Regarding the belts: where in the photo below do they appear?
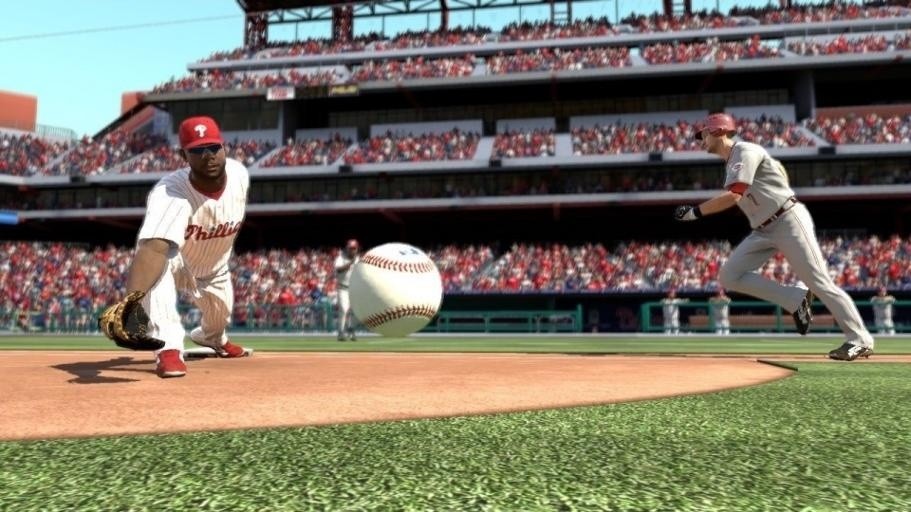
[759,197,797,230]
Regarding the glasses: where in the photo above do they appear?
[189,144,221,154]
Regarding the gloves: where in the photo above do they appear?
[675,206,704,220]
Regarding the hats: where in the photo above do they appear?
[346,240,359,248]
[179,117,224,149]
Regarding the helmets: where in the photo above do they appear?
[694,114,737,138]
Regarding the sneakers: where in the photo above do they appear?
[830,344,872,361]
[157,349,187,376]
[191,335,245,358]
[793,290,813,335]
